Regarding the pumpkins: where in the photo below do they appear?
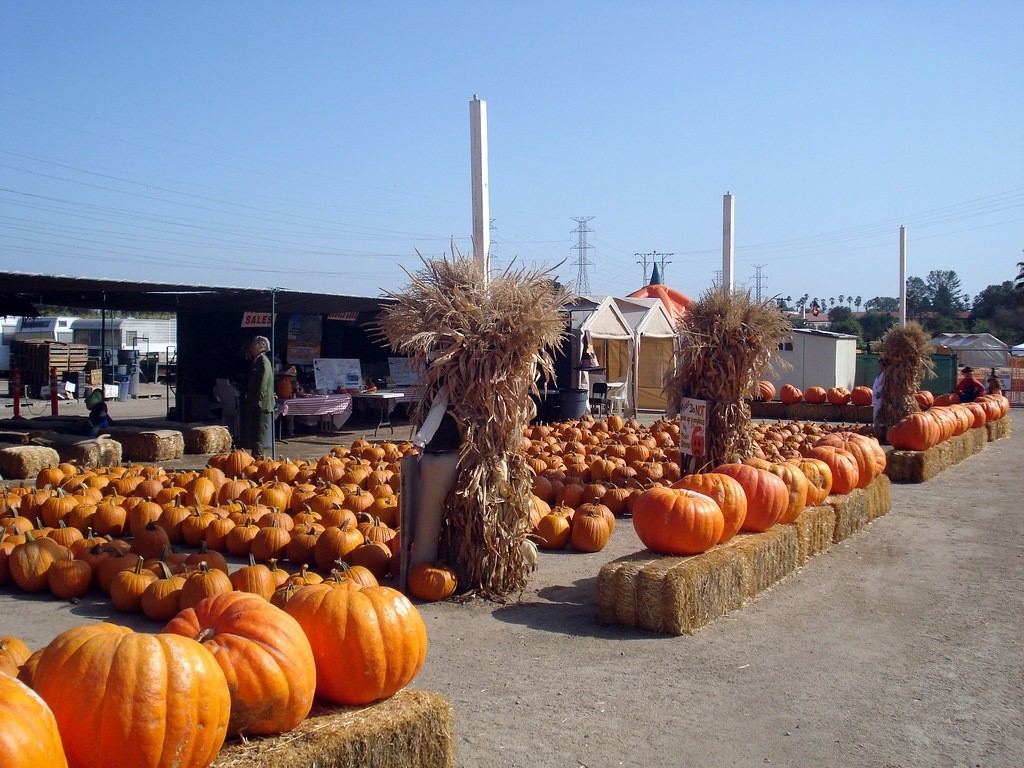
[0,431,459,768]
[522,410,888,554]
[750,379,875,406]
[885,390,1009,450]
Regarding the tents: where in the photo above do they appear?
[928,332,1008,367]
[571,294,679,421]
[1012,343,1024,356]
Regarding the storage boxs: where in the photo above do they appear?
[40,369,120,400]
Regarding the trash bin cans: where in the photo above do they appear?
[108,374,130,402]
[558,388,587,421]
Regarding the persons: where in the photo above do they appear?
[281,365,305,438]
[406,351,460,572]
[245,334,276,460]
[871,353,892,423]
[958,366,984,403]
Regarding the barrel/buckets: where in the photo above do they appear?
[558,388,588,422]
[98,349,139,401]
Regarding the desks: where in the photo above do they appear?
[352,392,404,437]
[275,394,353,444]
[357,388,425,424]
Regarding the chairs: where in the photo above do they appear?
[589,383,609,418]
[214,384,242,440]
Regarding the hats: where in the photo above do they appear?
[256,336,271,352]
[962,367,973,373]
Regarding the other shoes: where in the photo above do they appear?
[288,433,295,438]
